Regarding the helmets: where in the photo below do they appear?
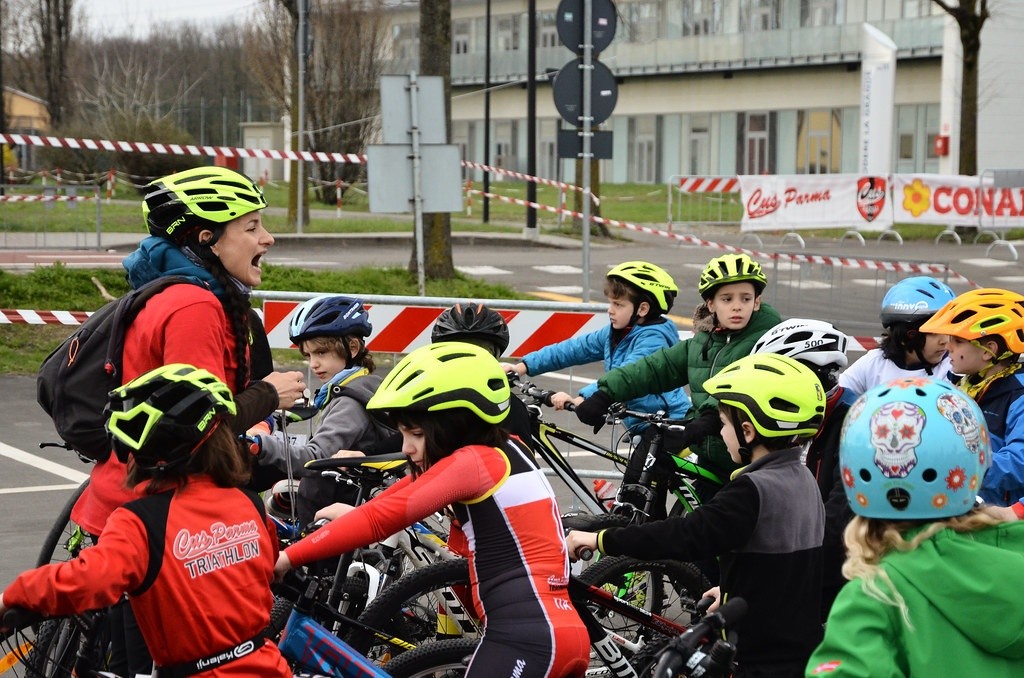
[837,378,994,519]
[287,294,372,344]
[365,342,511,424]
[880,278,956,327]
[917,287,1024,355]
[608,258,678,316]
[703,355,827,440]
[696,255,767,300]
[749,317,847,373]
[431,302,509,353]
[141,167,267,242]
[102,362,240,460]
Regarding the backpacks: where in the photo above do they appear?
[36,273,208,461]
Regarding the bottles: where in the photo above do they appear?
[593,478,618,509]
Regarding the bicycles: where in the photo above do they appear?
[0,370,749,678]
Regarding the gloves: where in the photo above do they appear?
[577,390,613,433]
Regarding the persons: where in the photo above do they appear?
[503,261,692,431]
[575,254,783,502]
[566,317,860,678]
[804,377,1024,678]
[241,297,401,477]
[297,303,533,576]
[69,166,305,678]
[272,342,590,678]
[837,276,1024,520]
[0,363,293,678]
